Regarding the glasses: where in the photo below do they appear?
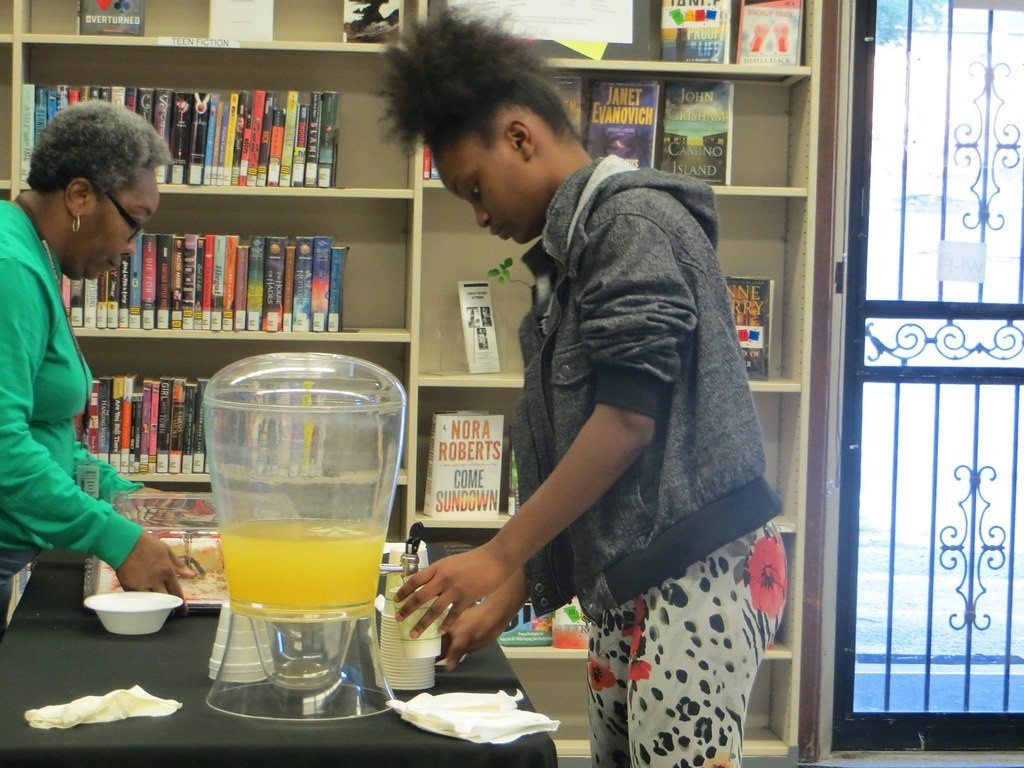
[95,182,146,245]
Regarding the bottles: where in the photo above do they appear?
[552,595,592,649]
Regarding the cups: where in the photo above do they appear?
[377,540,441,690]
[206,602,276,683]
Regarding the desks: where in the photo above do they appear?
[4,534,559,768]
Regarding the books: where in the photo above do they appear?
[1,0,799,664]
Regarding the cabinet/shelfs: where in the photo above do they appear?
[0,1,826,768]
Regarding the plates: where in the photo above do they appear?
[83,535,231,611]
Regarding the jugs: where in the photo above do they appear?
[202,352,405,719]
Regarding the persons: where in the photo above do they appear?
[373,0,788,767]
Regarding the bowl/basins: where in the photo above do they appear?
[83,592,183,635]
